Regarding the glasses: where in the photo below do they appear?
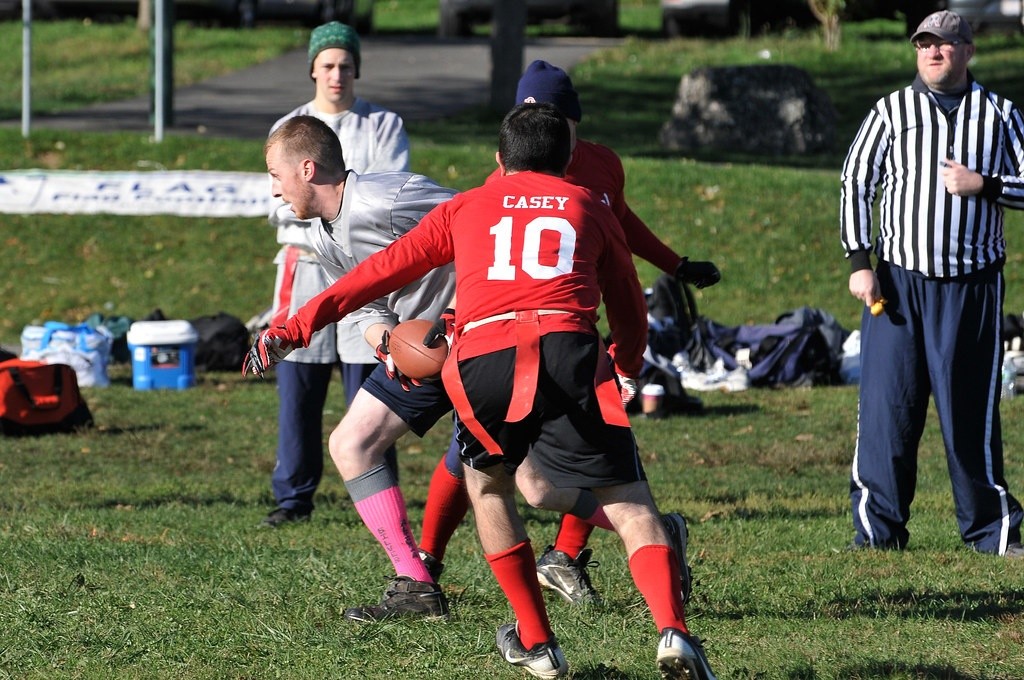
[915,38,968,51]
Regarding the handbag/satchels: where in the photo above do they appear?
[0,309,251,436]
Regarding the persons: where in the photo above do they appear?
[263,115,694,621]
[242,102,717,680]
[839,11,1024,556]
[264,20,410,529]
[413,59,721,605]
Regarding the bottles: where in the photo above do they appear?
[1000,356,1016,399]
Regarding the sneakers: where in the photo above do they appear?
[382,548,444,601]
[536,545,601,603]
[495,619,568,679]
[348,576,450,623]
[655,627,716,680]
[659,512,693,606]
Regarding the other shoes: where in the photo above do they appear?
[261,509,310,530]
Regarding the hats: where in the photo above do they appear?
[308,21,360,84]
[516,60,582,123]
[910,10,972,43]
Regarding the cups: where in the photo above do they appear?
[641,385,664,413]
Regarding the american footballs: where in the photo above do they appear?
[388,318,448,381]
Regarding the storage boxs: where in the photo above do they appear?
[127,320,198,391]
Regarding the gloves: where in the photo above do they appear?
[242,326,294,380]
[422,308,456,352]
[372,330,425,392]
[675,257,721,289]
[617,373,637,409]
